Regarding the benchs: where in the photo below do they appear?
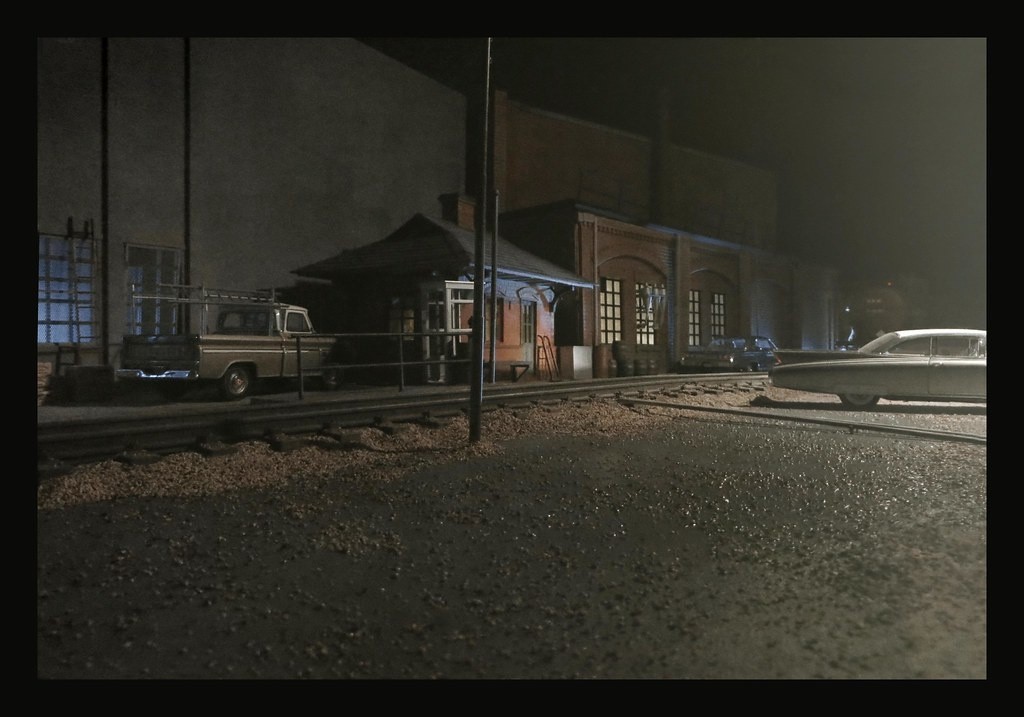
[484,360,532,383]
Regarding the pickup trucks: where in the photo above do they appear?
[120,299,348,402]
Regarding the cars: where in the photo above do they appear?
[767,328,987,413]
[699,336,780,374]
